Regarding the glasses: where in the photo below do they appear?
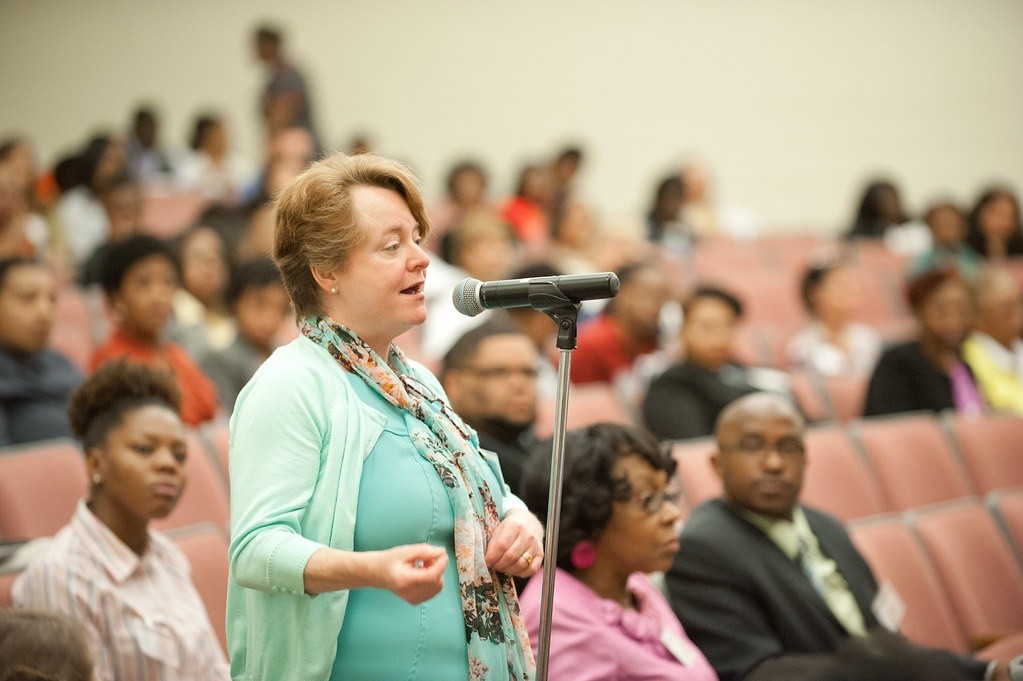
[467,363,541,380]
[614,488,681,513]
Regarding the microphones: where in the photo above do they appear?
[451,271,620,316]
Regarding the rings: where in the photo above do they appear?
[522,551,532,563]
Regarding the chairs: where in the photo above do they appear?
[2,201,1023,680]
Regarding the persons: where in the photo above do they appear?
[0,607,99,681]
[961,270,1021,418]
[150,225,239,366]
[860,265,982,421]
[787,260,886,383]
[14,356,231,681]
[82,234,220,425]
[1,255,86,451]
[195,251,295,409]
[556,251,672,386]
[249,26,324,206]
[225,149,547,681]
[2,103,1022,300]
[442,316,542,493]
[661,387,1023,681]
[511,419,719,681]
[636,287,755,440]
